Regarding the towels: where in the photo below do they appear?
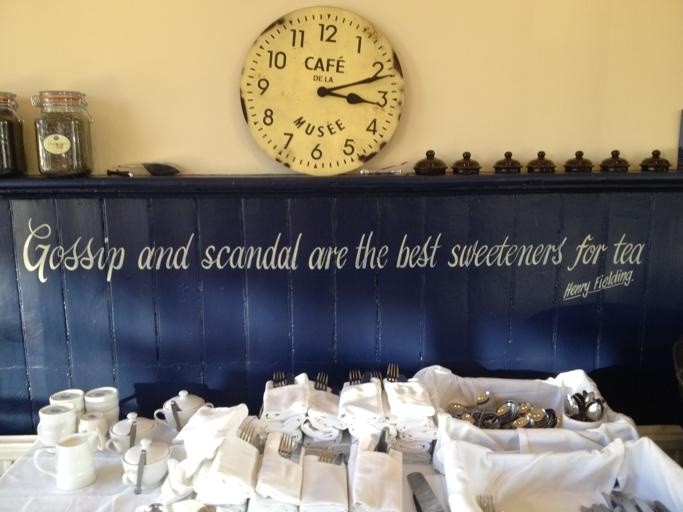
[173,374,437,512]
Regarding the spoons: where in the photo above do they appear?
[566,391,606,420]
[449,389,558,429]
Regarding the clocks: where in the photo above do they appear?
[239,5,404,177]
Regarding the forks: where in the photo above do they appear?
[240,425,336,467]
[271,362,404,391]
[481,495,495,511]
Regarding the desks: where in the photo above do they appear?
[0,170,683,432]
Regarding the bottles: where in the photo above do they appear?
[0,93,24,177]
[28,91,93,176]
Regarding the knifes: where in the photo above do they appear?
[584,491,671,512]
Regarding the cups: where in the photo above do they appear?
[119,437,169,491]
[108,413,157,450]
[31,383,118,451]
[561,411,600,429]
[154,387,215,430]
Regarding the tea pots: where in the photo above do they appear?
[31,431,98,491]
